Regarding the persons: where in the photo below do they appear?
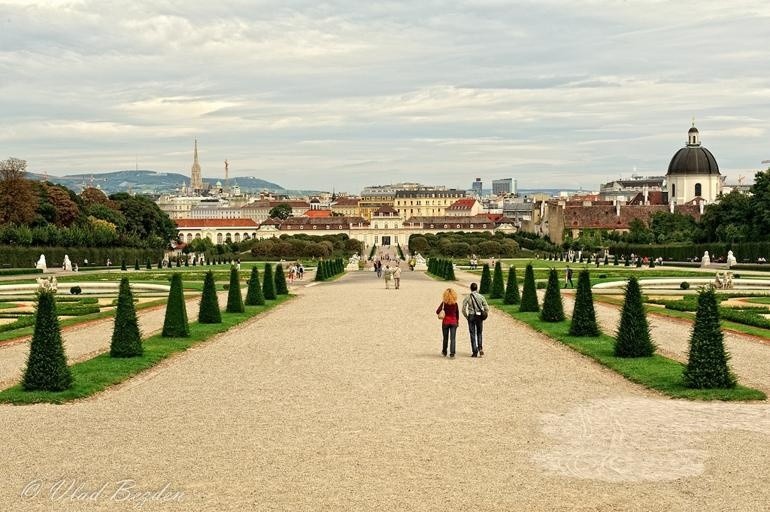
[473,259,477,269]
[462,283,490,357]
[371,250,404,290]
[435,288,459,357]
[563,265,574,288]
[236,257,240,270]
[469,257,474,270]
[488,256,492,266]
[490,256,495,266]
[634,255,663,266]
[288,262,305,284]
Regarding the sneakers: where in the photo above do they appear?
[442,347,485,359]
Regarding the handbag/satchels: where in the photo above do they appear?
[438,310,489,321]
